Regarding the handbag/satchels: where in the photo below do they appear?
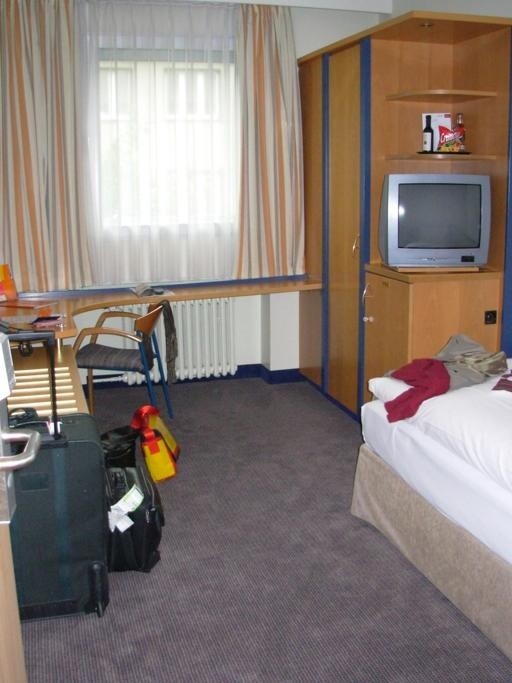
[99,423,165,573]
[130,403,182,483]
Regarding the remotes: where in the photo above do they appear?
[153,287,164,294]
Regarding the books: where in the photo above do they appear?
[0,298,59,310]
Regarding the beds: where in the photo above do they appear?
[350,360,512,661]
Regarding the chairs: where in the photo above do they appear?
[74,303,173,420]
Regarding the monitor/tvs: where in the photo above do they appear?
[376,173,493,267]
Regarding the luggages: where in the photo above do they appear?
[7,406,111,619]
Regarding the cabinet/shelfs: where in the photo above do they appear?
[297,10,511,422]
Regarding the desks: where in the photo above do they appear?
[1,278,324,415]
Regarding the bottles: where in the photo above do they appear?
[422,114,433,151]
[453,113,466,151]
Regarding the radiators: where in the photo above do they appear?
[120,297,237,385]
